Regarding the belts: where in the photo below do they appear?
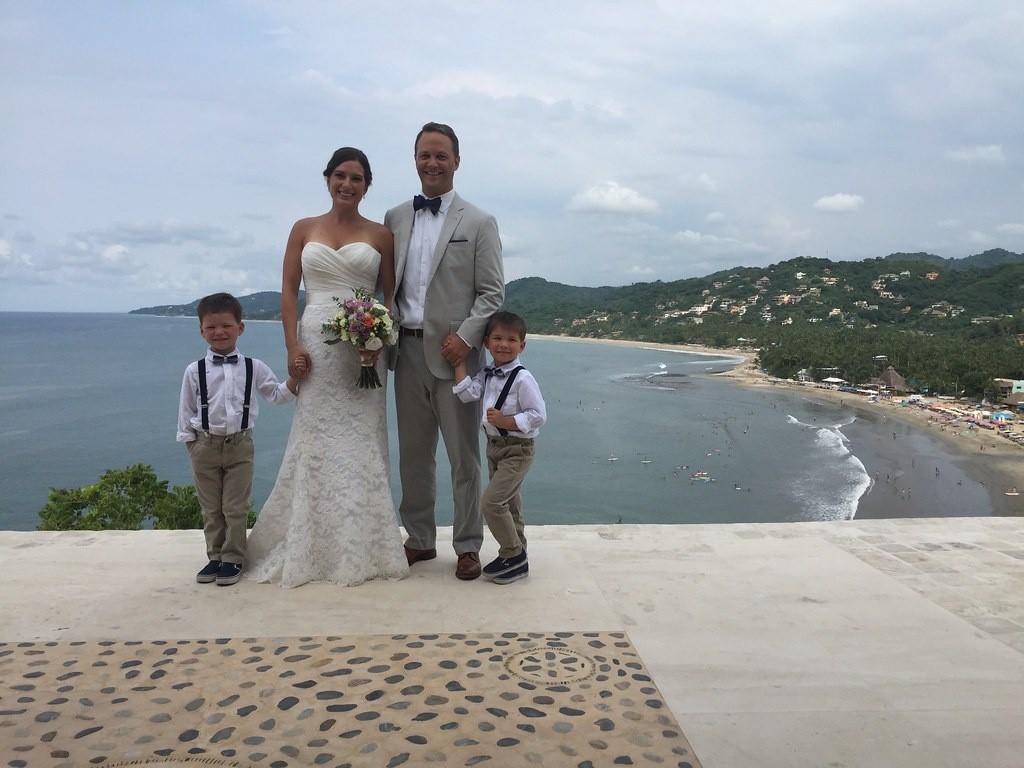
[399,326,423,338]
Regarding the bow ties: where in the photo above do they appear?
[213,355,238,366]
[413,194,442,216]
[484,367,505,378]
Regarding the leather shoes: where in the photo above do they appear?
[455,552,481,581]
[403,544,436,567]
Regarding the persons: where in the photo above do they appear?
[241,146,411,587]
[382,122,505,579]
[176,293,307,586]
[440,311,546,583]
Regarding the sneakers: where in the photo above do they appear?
[481,547,528,578]
[215,562,242,584]
[196,560,222,583]
[492,562,529,585]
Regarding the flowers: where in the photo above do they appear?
[321,283,404,390]
